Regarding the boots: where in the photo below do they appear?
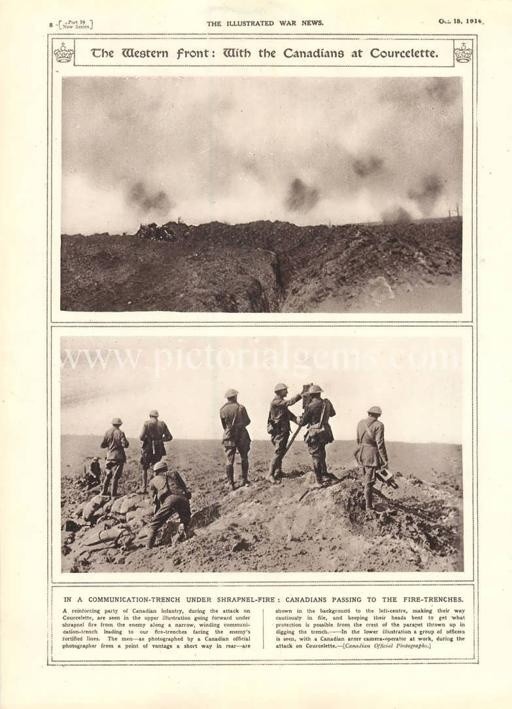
[265,460,285,485]
[134,470,148,495]
[307,459,326,488]
[240,461,251,487]
[224,464,236,494]
[364,487,376,511]
[97,478,120,494]
[145,525,158,551]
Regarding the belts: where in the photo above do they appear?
[358,441,377,448]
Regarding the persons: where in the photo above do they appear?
[145,461,195,550]
[353,405,389,518]
[98,417,129,497]
[220,388,251,491]
[263,383,307,484]
[299,384,336,485]
[135,408,173,494]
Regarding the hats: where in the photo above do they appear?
[147,409,162,419]
[151,460,169,474]
[307,385,325,396]
[366,404,385,417]
[272,383,290,393]
[223,389,241,399]
[109,416,124,427]
